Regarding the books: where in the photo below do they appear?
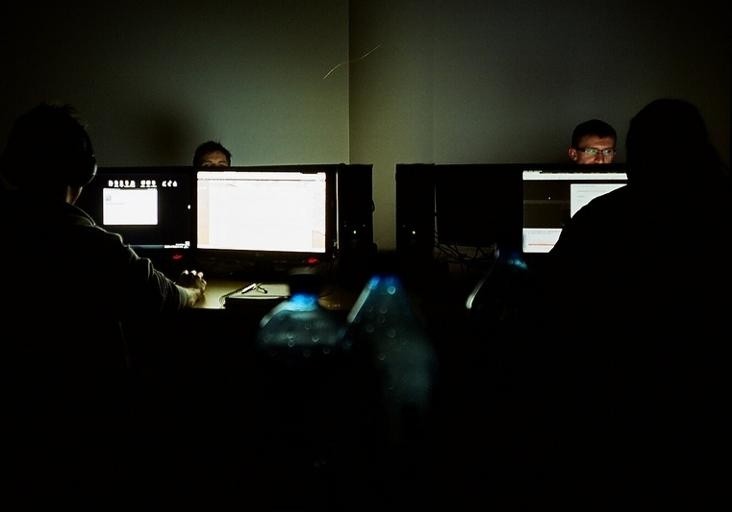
[224,282,291,310]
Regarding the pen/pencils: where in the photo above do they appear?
[241,283,256,293]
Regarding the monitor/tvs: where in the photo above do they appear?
[74,167,192,269]
[518,168,628,255]
[436,163,549,259]
[192,165,332,269]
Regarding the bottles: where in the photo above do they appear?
[261,268,338,361]
[339,253,431,420]
[461,234,531,331]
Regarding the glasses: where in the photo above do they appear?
[576,146,615,156]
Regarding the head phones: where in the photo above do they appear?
[0,110,98,187]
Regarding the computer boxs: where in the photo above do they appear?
[338,164,373,250]
[395,163,436,258]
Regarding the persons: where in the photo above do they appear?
[540,92,732,281]
[566,118,620,167]
[1,100,210,318]
[193,140,234,169]
